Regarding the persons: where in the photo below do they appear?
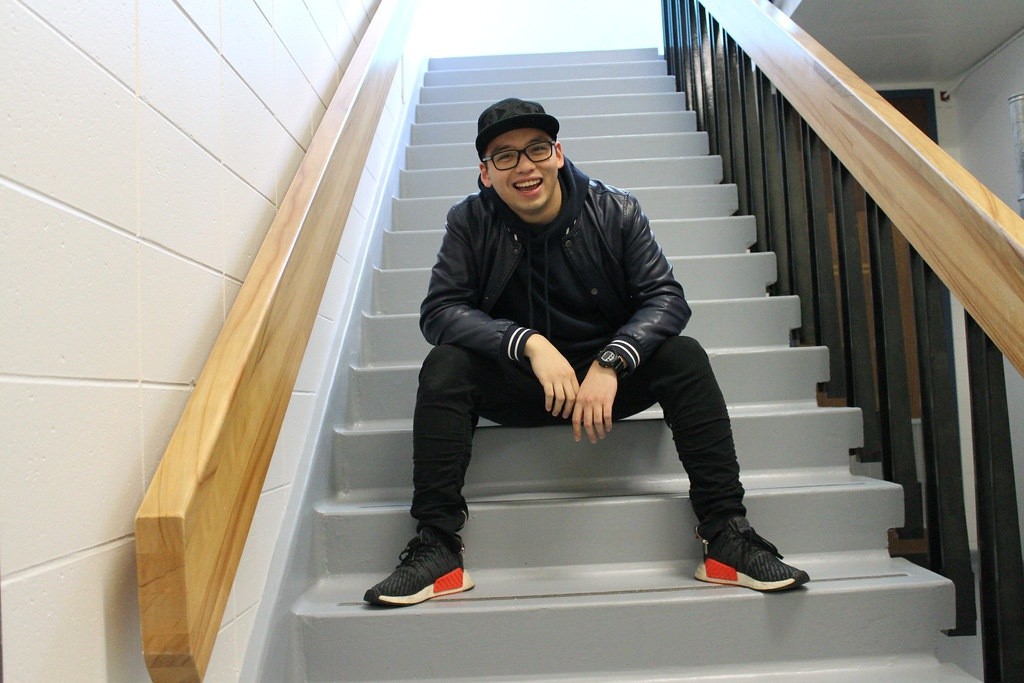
[362,96,811,605]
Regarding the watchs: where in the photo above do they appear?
[595,347,629,379]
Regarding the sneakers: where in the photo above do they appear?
[364,527,475,604]
[693,515,810,590]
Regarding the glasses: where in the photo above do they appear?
[482,141,556,171]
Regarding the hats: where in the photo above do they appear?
[476,98,560,155]
